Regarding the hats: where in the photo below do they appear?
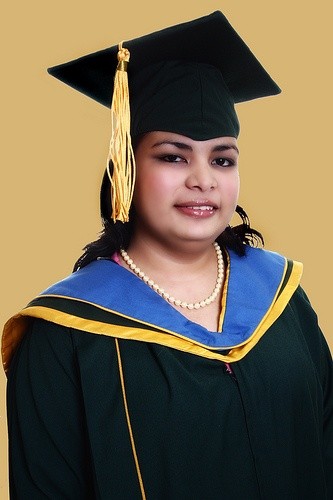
[47,11,282,224]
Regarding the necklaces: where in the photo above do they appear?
[119,242,225,310]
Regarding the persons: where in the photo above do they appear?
[0,10,333,500]
